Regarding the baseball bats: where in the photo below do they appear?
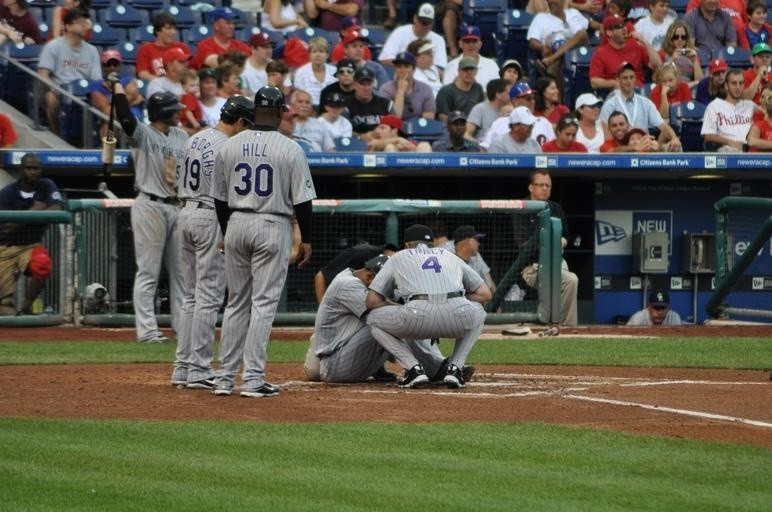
[103,83,115,184]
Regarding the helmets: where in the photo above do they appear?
[221,94,255,127]
[255,83,290,112]
[364,255,391,271]
[147,92,186,112]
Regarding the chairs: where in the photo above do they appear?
[612,315,631,326]
[0,0,772,152]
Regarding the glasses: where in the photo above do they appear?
[673,35,687,40]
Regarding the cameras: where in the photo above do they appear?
[681,49,689,55]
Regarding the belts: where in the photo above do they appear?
[149,194,177,204]
[411,291,463,300]
[182,198,213,210]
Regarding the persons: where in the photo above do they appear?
[172,86,318,397]
[0,1,772,153]
[107,53,195,345]
[626,289,682,326]
[513,170,580,328]
[307,220,495,390]
[0,154,64,316]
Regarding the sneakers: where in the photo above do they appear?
[171,376,233,394]
[397,364,429,387]
[240,382,280,398]
[428,360,476,389]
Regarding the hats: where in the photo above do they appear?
[460,26,482,40]
[448,57,604,126]
[101,49,121,64]
[709,58,728,74]
[617,61,634,78]
[627,128,648,140]
[751,42,771,56]
[251,32,274,47]
[63,8,92,22]
[30,246,52,277]
[210,8,240,25]
[604,13,626,30]
[402,223,434,241]
[325,3,436,130]
[454,226,486,242]
[648,290,670,307]
[162,48,192,64]
